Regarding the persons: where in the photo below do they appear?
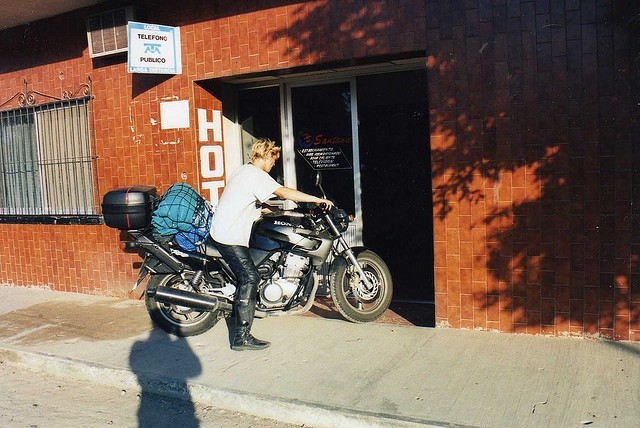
[209,137,335,351]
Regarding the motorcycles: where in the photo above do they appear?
[101,170,394,338]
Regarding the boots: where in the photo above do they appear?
[231,298,271,351]
